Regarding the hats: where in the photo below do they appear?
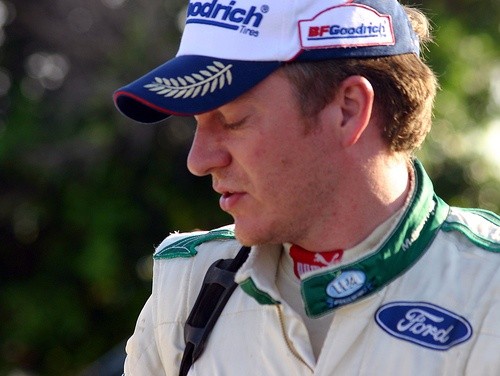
[112,0,422,121]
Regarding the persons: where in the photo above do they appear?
[113,0,500,376]
